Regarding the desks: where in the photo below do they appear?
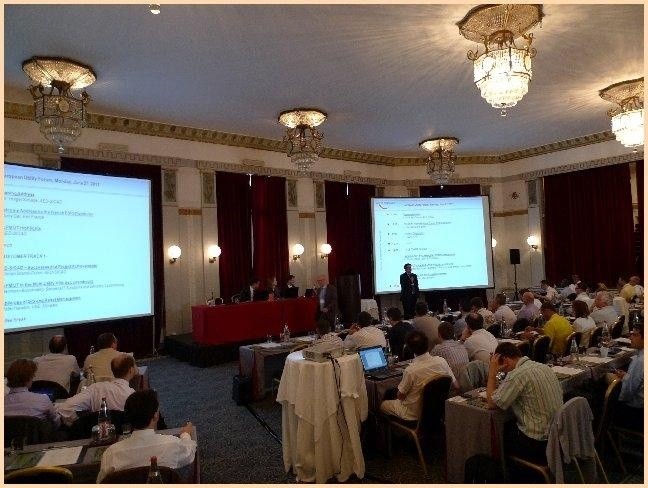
[275,346,370,484]
[188,297,323,343]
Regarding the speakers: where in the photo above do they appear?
[509,248,520,264]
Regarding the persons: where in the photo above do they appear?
[54,354,140,428]
[31,334,81,393]
[4,358,61,427]
[283,273,299,297]
[238,273,261,300]
[315,274,643,376]
[97,387,197,483]
[398,263,422,318]
[485,341,564,460]
[265,275,280,298]
[617,323,643,412]
[379,330,459,422]
[82,332,135,385]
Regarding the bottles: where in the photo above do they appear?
[600,320,611,338]
[283,323,291,342]
[147,455,164,483]
[442,298,448,312]
[98,395,113,442]
[569,335,580,361]
[500,315,508,336]
[89,345,95,354]
[86,365,96,386]
[334,316,341,332]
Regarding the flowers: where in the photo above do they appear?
[386,372,453,477]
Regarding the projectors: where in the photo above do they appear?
[301,340,344,362]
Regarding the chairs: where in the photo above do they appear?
[444,299,645,485]
[233,299,445,435]
[4,358,201,484]
[205,296,223,304]
[231,294,240,304]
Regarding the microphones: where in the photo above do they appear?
[211,291,213,299]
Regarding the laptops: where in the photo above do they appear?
[355,344,402,380]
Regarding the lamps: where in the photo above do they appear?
[207,244,222,262]
[293,243,304,260]
[319,242,332,258]
[598,74,645,155]
[455,4,543,117]
[276,106,327,172]
[420,136,456,190]
[168,243,181,263]
[22,55,96,152]
[526,232,540,251]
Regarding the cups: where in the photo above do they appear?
[121,423,132,437]
[600,347,608,357]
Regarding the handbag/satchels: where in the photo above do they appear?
[464,454,504,484]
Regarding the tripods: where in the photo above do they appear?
[511,264,520,302]
